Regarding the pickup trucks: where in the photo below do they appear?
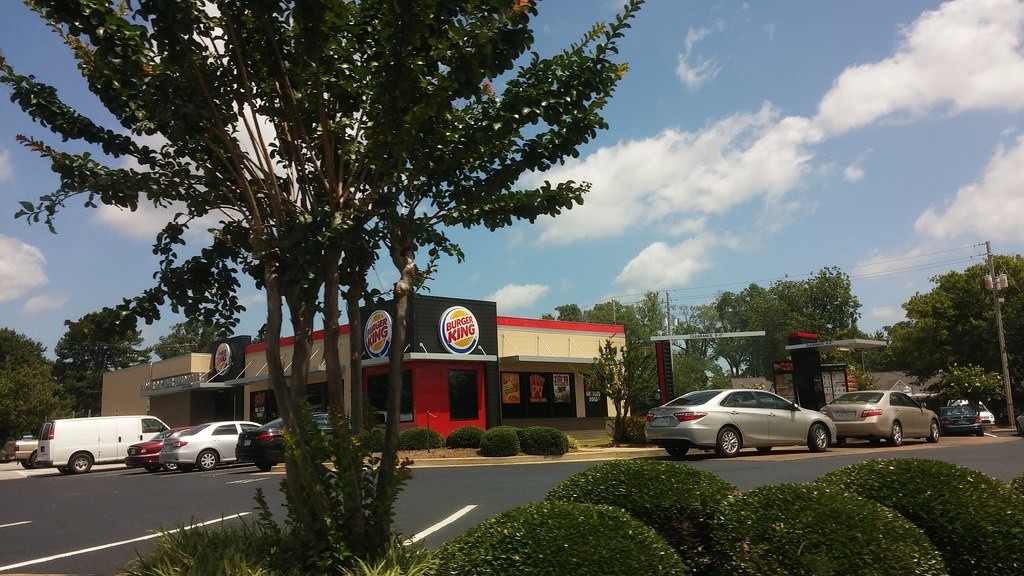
[4,437,47,468]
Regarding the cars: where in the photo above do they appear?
[236,412,352,470]
[820,390,943,447]
[124,425,198,472]
[159,421,263,471]
[1016,408,1024,437]
[641,389,839,457]
[950,401,996,427]
[932,406,985,438]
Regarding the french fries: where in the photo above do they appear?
[530,374,545,386]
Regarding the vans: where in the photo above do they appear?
[35,414,170,475]
